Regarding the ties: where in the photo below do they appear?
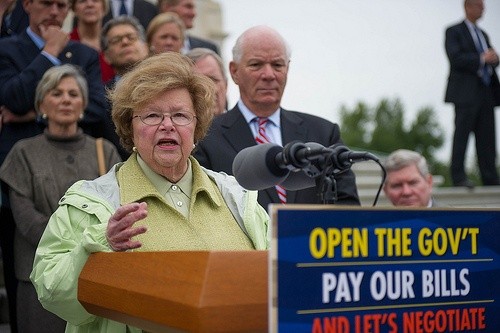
[475,29,490,85]
[256,117,288,204]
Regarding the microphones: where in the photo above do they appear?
[232,141,324,191]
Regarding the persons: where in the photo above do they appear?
[381,150,434,208]
[445,0,500,186]
[1,63,122,331]
[30,51,278,333]
[0,0,230,160]
[193,25,361,205]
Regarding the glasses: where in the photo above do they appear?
[129,104,199,127]
[103,34,138,52]
